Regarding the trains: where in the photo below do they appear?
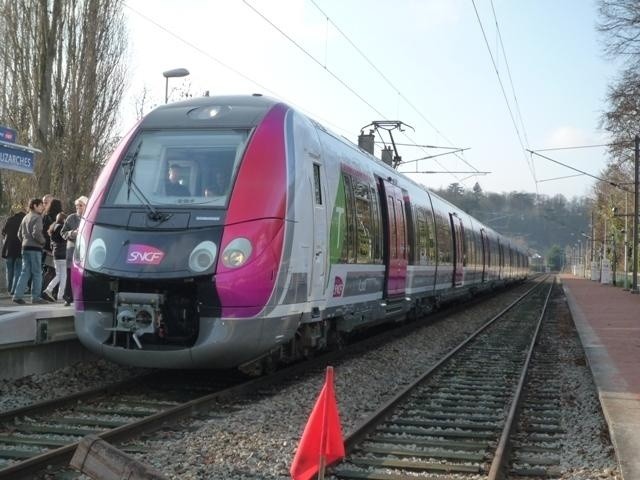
[70,93,546,377]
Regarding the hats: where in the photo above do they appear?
[74,196,88,205]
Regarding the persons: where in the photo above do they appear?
[158,163,191,196]
[2,193,89,307]
[203,183,218,197]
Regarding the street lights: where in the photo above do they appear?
[163,67,190,104]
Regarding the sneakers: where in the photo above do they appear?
[7,288,71,306]
